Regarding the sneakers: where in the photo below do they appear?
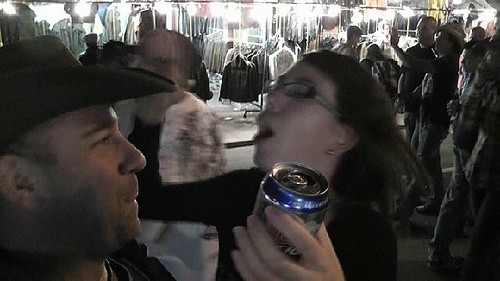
[427,246,465,271]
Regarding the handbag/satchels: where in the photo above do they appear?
[451,112,480,151]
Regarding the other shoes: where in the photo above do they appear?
[388,200,419,222]
[416,197,443,215]
[455,230,470,241]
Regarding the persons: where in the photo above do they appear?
[0,35,178,281]
[111,0,500,280]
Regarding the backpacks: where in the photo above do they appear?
[362,58,400,97]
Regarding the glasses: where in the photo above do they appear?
[263,78,343,116]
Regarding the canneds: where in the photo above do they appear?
[251,162,329,262]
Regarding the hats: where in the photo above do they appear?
[0,34,178,155]
[437,21,466,48]
